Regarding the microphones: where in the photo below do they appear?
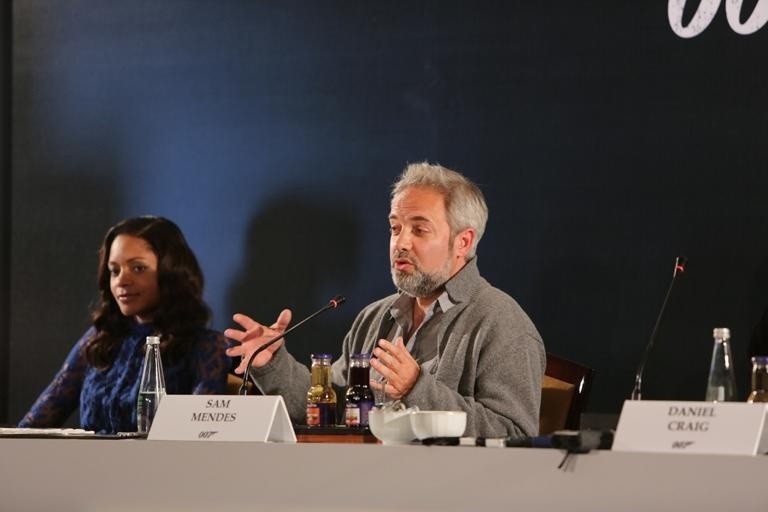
[631,254,689,400]
[237,294,347,396]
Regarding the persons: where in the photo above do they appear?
[221,160,546,439]
[16,214,233,435]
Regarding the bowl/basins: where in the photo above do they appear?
[366,410,419,448]
[406,410,468,446]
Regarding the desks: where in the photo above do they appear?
[1,425,767,512]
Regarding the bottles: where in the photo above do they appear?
[134,334,169,437]
[702,325,738,406]
[303,351,339,432]
[744,355,767,404]
[343,352,377,434]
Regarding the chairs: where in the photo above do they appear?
[538,352,597,436]
